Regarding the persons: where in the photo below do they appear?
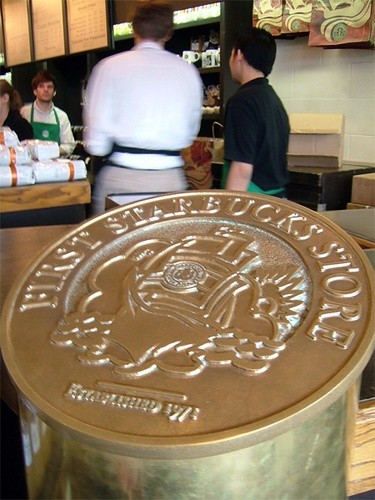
[20,72,76,160]
[221,26,291,201]
[82,3,203,215]
[0,80,34,141]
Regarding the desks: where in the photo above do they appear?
[0,176,92,227]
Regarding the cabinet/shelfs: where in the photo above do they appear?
[1,209,373,500]
[287,164,374,213]
[112,0,255,163]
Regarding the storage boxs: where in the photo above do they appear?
[351,173,375,207]
[347,203,374,210]
[287,111,345,168]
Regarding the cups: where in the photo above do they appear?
[182,48,221,68]
